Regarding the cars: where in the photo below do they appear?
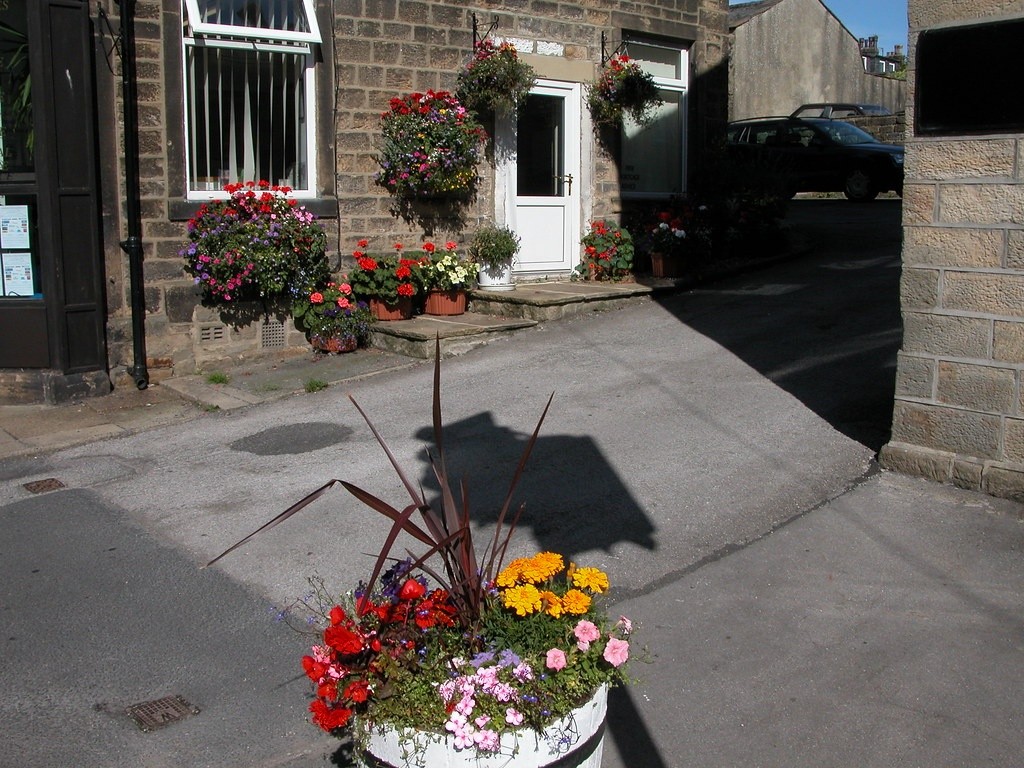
[790,103,894,119]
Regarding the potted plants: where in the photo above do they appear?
[470,224,520,291]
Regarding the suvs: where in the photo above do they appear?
[726,115,907,201]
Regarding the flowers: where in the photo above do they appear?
[635,203,689,246]
[588,53,662,128]
[574,220,634,272]
[200,330,634,765]
[455,39,539,116]
[414,243,479,294]
[294,275,372,359]
[179,181,328,311]
[372,90,487,205]
[347,241,418,305]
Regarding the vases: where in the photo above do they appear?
[368,297,413,320]
[310,327,358,353]
[422,288,465,315]
[650,247,688,277]
[353,675,609,768]
